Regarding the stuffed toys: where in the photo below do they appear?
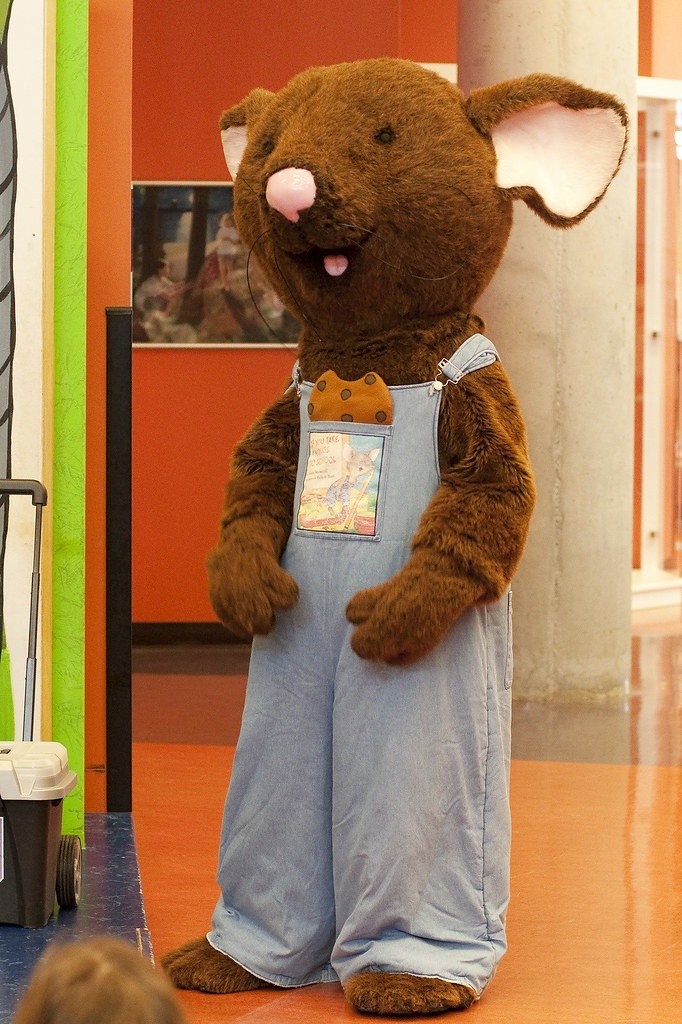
[161,58,628,1012]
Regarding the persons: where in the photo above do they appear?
[13,938,186,1024]
[134,258,197,343]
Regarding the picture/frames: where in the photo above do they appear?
[129,178,300,350]
